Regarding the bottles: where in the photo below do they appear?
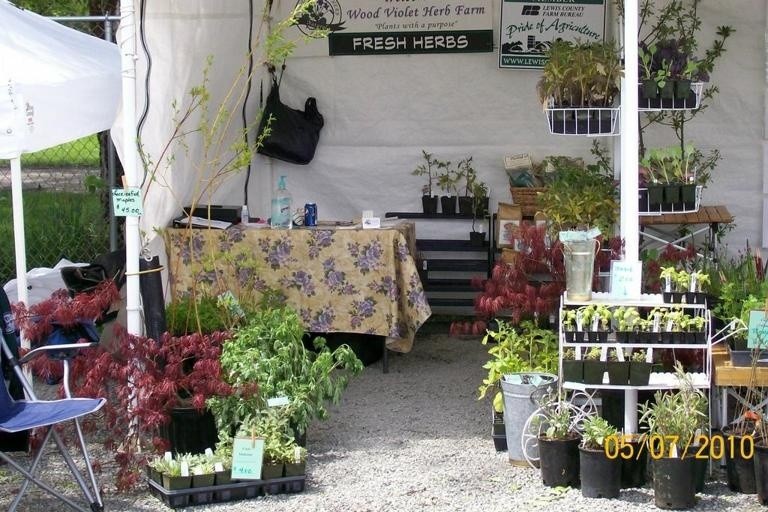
[241,205,249,225]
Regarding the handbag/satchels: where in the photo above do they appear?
[253,80,327,166]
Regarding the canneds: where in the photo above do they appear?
[305,203,317,226]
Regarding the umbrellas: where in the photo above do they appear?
[0,0,124,403]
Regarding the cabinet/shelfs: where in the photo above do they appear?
[384,211,492,330]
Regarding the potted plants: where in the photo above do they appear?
[643,393,768,511]
[615,436,648,490]
[530,408,580,486]
[578,416,624,498]
[540,4,734,133]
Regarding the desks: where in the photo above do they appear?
[170,218,431,374]
